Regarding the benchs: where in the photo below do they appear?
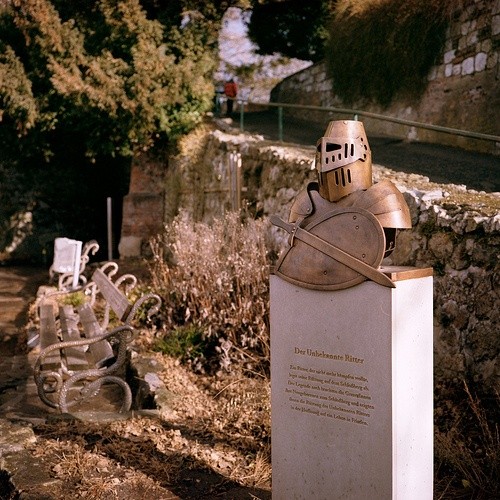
[48,239,100,292]
[34,261,162,413]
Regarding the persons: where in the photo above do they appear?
[285,119,412,264]
[225,79,236,115]
